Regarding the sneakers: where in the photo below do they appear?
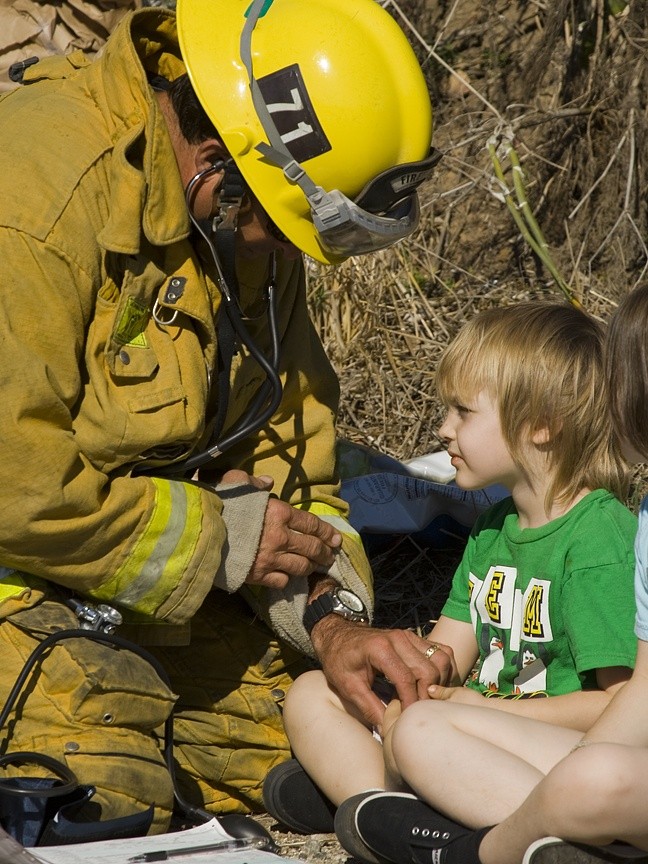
[333,788,470,864]
[522,835,648,864]
[264,758,339,835]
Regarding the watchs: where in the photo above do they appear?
[304,586,370,635]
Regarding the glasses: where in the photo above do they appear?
[304,187,426,259]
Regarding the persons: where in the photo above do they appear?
[264,301,640,837]
[334,282,647,863]
[0,0,457,842]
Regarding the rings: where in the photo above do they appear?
[425,645,443,661]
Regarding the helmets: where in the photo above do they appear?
[172,0,444,267]
